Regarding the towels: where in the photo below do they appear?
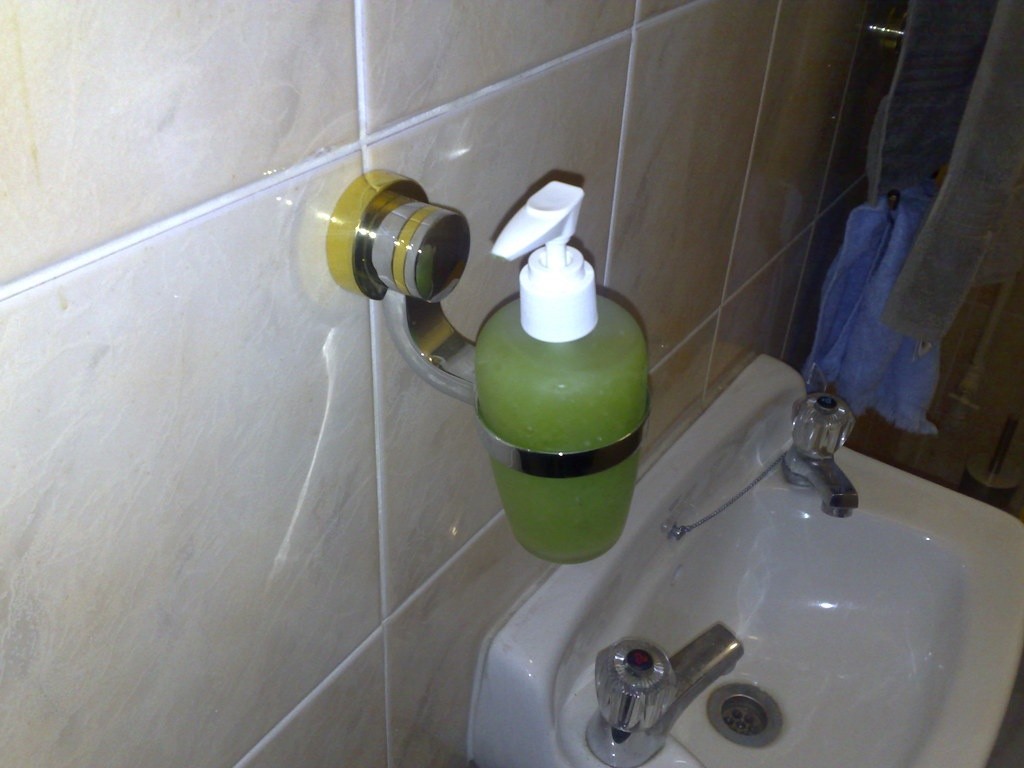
[801,184,942,436]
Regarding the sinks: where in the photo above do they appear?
[603,435,1024,768]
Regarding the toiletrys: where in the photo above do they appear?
[473,179,652,564]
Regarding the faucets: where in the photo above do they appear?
[781,392,860,520]
[584,622,745,768]
